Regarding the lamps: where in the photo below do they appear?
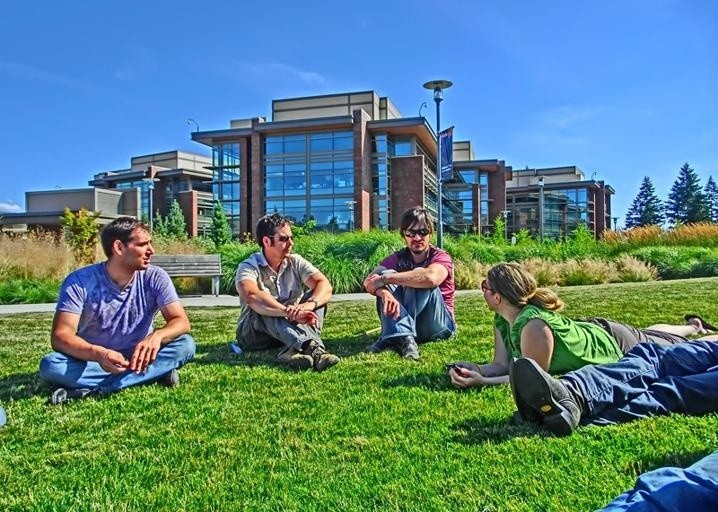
[589,169,598,180]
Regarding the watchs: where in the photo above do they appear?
[307,297,318,311]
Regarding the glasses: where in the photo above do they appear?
[402,230,429,236]
[482,280,492,291]
[269,236,293,241]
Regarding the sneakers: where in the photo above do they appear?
[165,370,179,386]
[275,351,314,368]
[363,340,385,353]
[396,336,419,360]
[304,340,340,372]
[53,388,67,404]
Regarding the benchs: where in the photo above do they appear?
[148,253,226,297]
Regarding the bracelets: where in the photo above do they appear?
[379,274,384,280]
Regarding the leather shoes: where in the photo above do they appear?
[509,356,540,425]
[513,358,581,437]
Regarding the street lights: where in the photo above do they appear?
[501,210,511,238]
[142,177,160,232]
[535,174,546,243]
[610,217,619,232]
[423,79,453,254]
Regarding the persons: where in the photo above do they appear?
[235,212,340,374]
[361,204,458,362]
[590,447,718,512]
[444,260,716,391]
[38,217,197,406]
[506,340,716,440]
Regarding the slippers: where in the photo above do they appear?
[685,315,718,333]
